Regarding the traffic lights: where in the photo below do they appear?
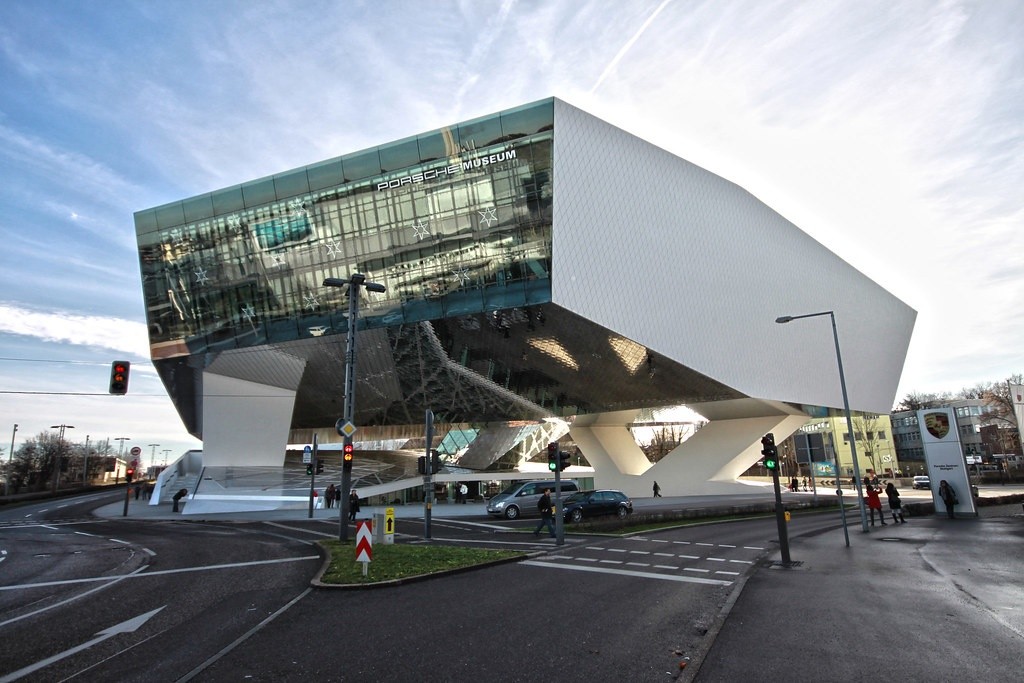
[109,361,131,396]
[760,436,777,472]
[559,451,571,472]
[343,444,353,473]
[125,469,133,482]
[432,450,442,474]
[307,464,313,475]
[316,457,324,475]
[548,444,558,471]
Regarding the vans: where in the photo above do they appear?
[486,480,580,520]
[309,326,330,337]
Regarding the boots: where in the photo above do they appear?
[892,513,899,523]
[898,513,907,524]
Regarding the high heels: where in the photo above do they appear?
[881,522,887,526]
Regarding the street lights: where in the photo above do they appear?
[323,273,386,542]
[149,444,160,481]
[50,424,76,488]
[163,449,172,469]
[775,310,869,533]
[115,437,130,486]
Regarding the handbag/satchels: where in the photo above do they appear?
[863,497,869,505]
[954,499,959,505]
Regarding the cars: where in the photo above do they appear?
[911,476,930,490]
[552,490,633,524]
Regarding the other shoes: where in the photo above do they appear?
[550,535,556,538]
[533,531,540,536]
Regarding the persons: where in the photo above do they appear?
[866,485,887,526]
[888,468,903,478]
[852,475,858,490]
[324,484,342,509]
[863,474,870,492]
[534,488,556,538]
[872,475,879,490]
[653,481,661,497]
[939,480,956,519]
[455,483,468,504]
[885,483,908,523]
[134,482,156,500]
[348,490,360,521]
[792,476,813,492]
[173,489,187,512]
